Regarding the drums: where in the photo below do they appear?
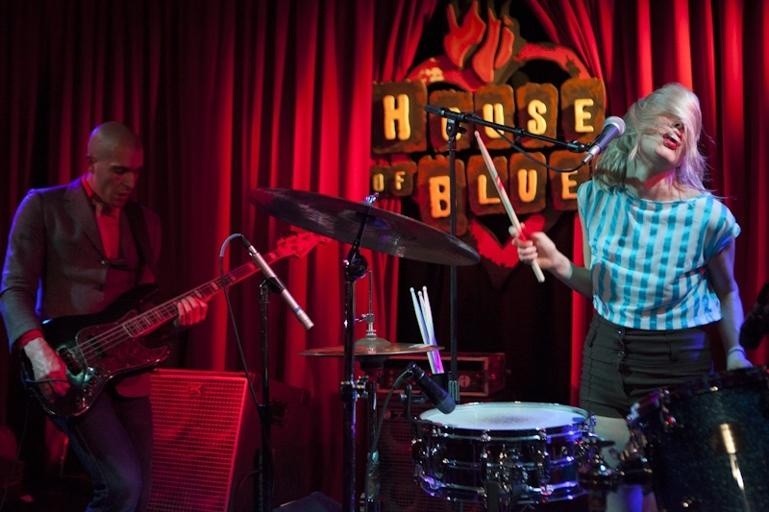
[623,367,769,511]
[413,401,595,504]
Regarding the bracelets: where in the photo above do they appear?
[724,345,745,354]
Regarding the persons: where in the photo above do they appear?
[503,82,756,512]
[0,121,210,509]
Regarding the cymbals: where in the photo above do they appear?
[300,337,446,357]
[247,188,480,266]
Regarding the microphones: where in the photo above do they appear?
[581,115,627,165]
[407,361,456,414]
[242,237,314,330]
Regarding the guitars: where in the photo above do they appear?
[20,232,330,417]
